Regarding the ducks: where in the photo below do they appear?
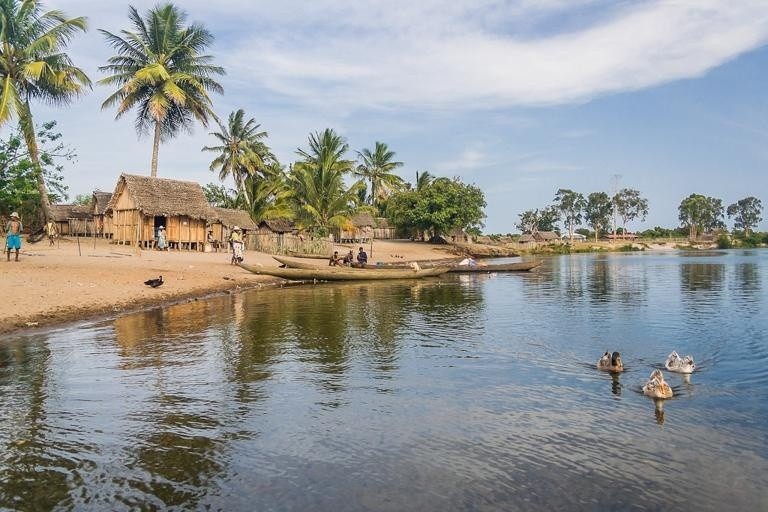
[144,275,164,288]
[642,368,673,398]
[664,350,695,374]
[597,350,623,373]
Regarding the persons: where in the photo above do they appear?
[155,225,167,251]
[230,225,244,266]
[342,249,354,268]
[357,246,367,265]
[329,251,342,267]
[206,228,218,252]
[237,229,246,252]
[3,211,24,262]
[42,218,57,247]
